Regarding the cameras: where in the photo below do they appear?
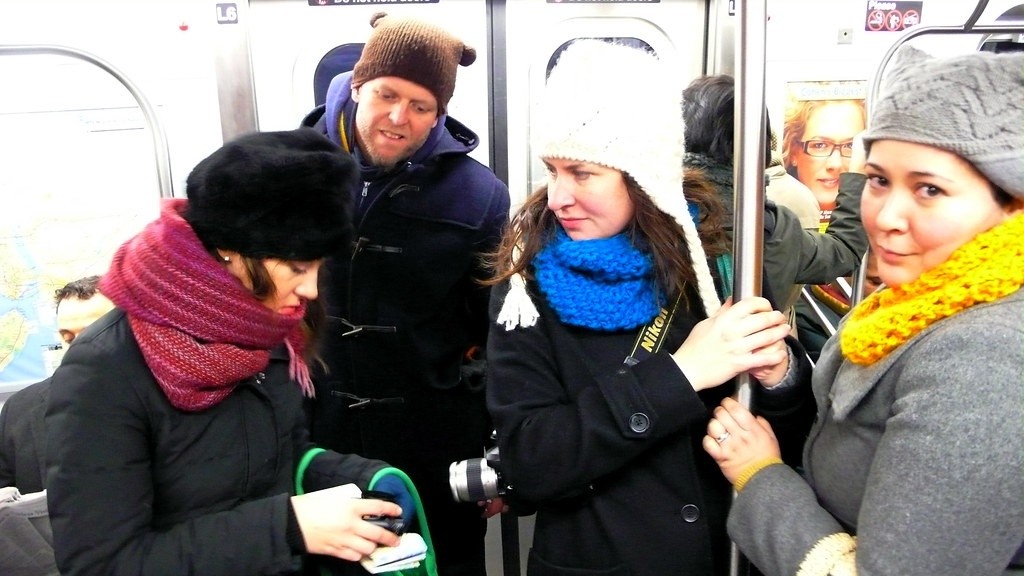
[450,428,504,506]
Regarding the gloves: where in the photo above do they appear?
[369,474,414,534]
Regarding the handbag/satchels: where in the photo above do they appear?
[294,447,438,576]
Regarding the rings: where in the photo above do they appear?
[715,429,729,444]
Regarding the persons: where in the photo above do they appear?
[783,100,867,234]
[299,11,508,576]
[681,75,869,341]
[704,42,1024,576]
[487,41,819,576]
[0,275,116,496]
[43,126,415,576]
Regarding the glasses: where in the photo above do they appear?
[796,140,853,158]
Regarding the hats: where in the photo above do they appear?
[862,43,1024,202]
[300,43,366,126]
[185,127,356,261]
[351,12,476,119]
[496,39,721,332]
